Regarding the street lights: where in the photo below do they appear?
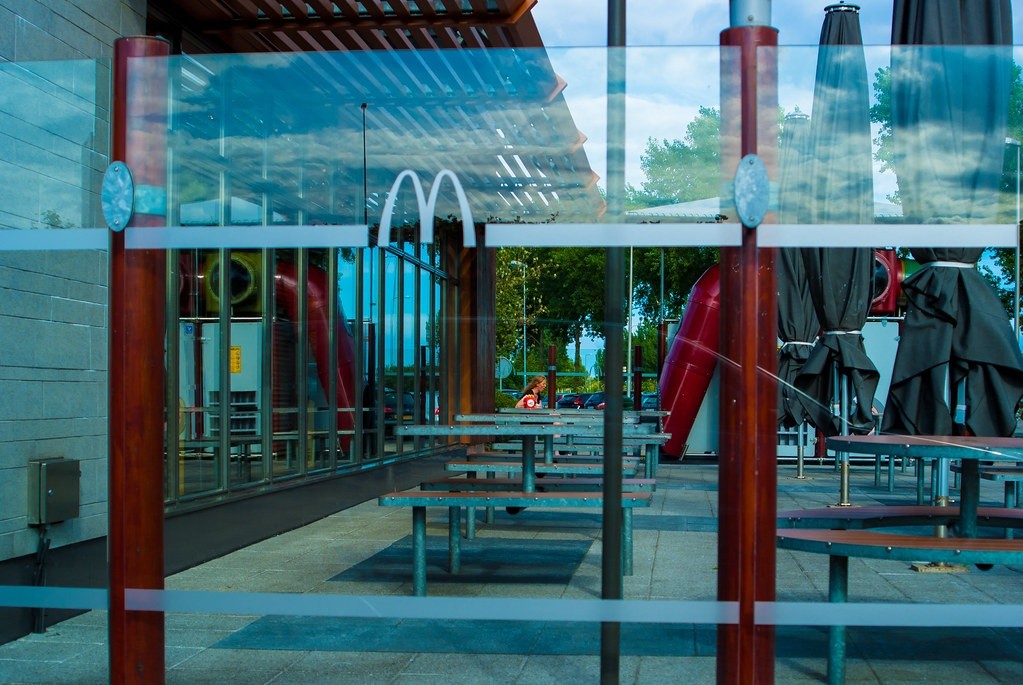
[511,260,528,391]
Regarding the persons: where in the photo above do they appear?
[515,375,547,408]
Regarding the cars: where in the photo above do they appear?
[377,391,417,436]
[423,391,440,425]
[584,390,634,409]
[542,393,593,409]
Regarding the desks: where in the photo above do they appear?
[395,408,672,437]
[829,436,1023,538]
[169,408,371,417]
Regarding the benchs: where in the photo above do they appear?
[776,506,1023,685]
[378,434,672,599]
[163,430,378,498]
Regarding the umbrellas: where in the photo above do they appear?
[778,6,879,508]
[888,5,1023,566]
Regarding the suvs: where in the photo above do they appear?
[641,394,659,411]
